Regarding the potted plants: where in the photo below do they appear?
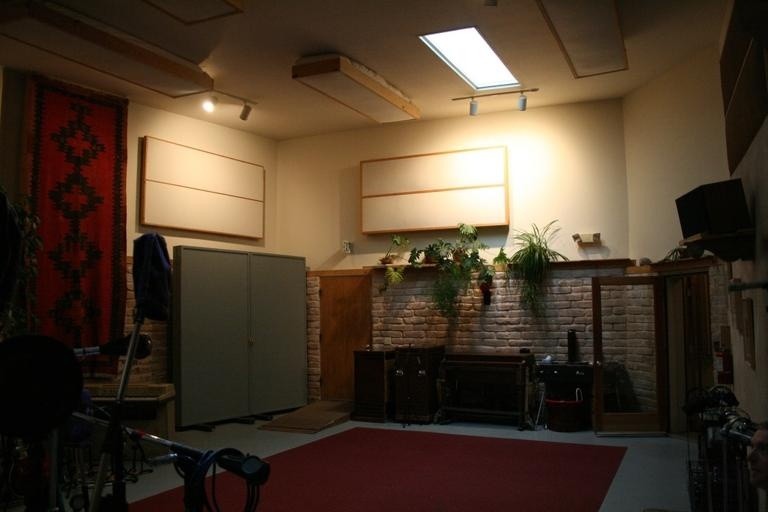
[423,237,451,265]
[431,222,496,327]
[380,234,409,266]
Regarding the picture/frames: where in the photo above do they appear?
[359,145,511,235]
[141,134,266,241]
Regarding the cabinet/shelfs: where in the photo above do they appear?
[353,346,397,421]
[390,345,446,425]
[435,353,539,431]
[171,243,309,429]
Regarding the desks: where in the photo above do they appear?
[536,361,624,429]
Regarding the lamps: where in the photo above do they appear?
[449,85,540,115]
[201,86,258,121]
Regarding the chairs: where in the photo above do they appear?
[533,374,588,431]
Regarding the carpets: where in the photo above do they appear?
[127,428,630,512]
